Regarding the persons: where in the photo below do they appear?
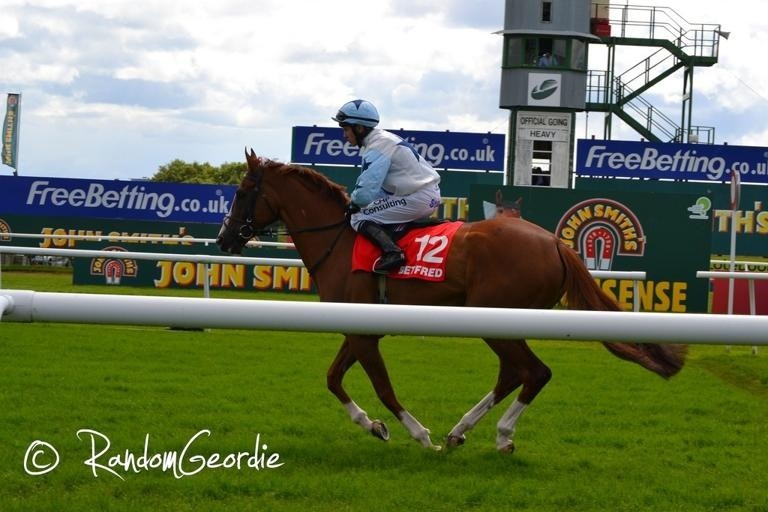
[331,100,440,270]
[539,49,557,66]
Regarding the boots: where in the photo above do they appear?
[360,222,405,270]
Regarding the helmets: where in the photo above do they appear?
[331,100,379,128]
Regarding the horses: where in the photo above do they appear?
[215,146,690,454]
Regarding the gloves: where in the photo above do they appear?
[344,202,360,227]
